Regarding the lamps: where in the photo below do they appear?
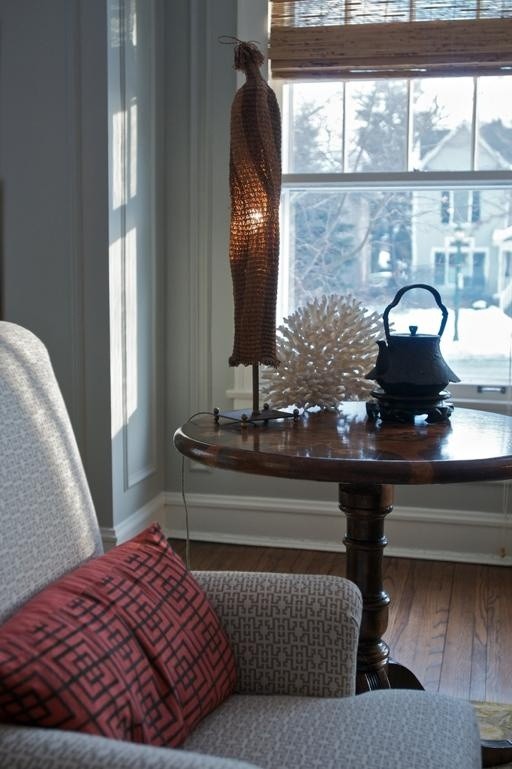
[214,33,301,426]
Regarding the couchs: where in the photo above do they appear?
[1,320,484,769]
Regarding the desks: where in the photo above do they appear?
[173,400,511,768]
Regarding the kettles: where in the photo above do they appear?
[364,284,460,433]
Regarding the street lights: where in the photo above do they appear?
[453,222,465,340]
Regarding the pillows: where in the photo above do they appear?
[2,521,238,749]
